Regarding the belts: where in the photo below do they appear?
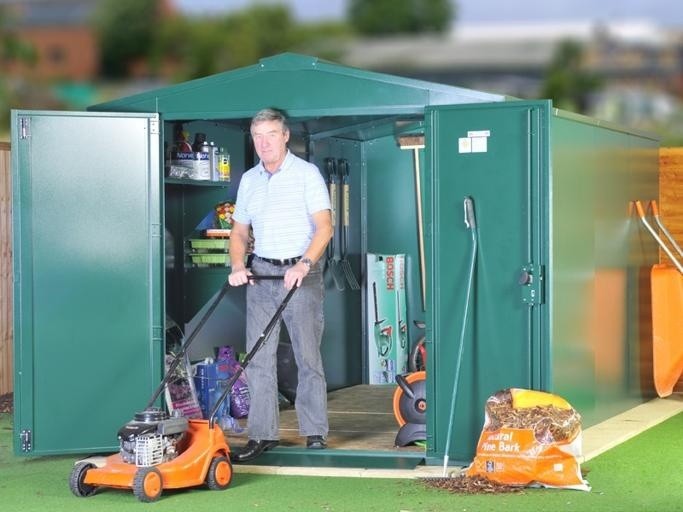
[252,252,304,266]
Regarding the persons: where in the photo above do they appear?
[226,107,337,462]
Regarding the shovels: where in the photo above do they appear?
[327,157,350,290]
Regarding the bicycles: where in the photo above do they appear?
[409,319,427,373]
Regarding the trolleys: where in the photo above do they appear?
[633,199,683,398]
[69,274,300,504]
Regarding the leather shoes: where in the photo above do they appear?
[233,436,283,461]
[306,434,331,447]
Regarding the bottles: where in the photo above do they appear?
[166,130,218,181]
[217,147,232,183]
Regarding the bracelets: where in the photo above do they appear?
[298,257,312,269]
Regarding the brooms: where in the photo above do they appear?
[396,135,427,313]
